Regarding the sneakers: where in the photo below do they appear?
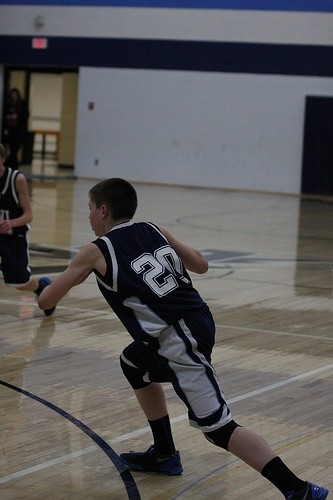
[286,480,328,500]
[120,445,184,476]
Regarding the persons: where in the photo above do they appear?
[4,88,28,171]
[38,178,329,500]
[0,132,55,316]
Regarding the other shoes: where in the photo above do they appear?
[36,277,55,317]
